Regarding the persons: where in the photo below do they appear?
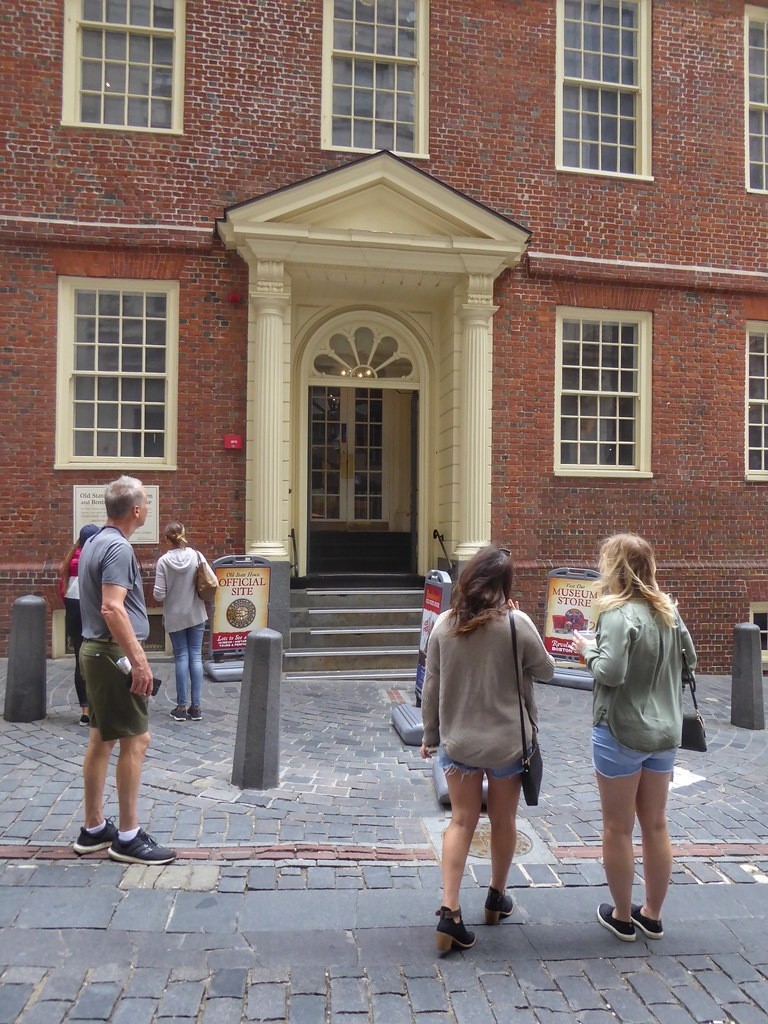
[74,474,177,865]
[420,548,555,954]
[570,533,698,943]
[60,523,101,726]
[153,521,219,720]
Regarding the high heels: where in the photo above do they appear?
[485,887,514,922]
[436,906,477,951]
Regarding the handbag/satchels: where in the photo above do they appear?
[680,714,707,752]
[522,743,543,806]
[196,550,217,601]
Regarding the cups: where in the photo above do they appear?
[577,630,599,664]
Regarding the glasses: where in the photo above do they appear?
[479,546,512,557]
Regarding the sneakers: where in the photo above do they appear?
[74,817,118,853]
[187,707,202,721]
[170,705,186,721]
[108,827,177,864]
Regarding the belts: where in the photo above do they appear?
[91,639,144,645]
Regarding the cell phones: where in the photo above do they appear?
[125,671,162,696]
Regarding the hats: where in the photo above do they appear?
[81,524,99,541]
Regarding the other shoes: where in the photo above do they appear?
[631,903,664,938]
[80,715,89,726]
[598,903,636,942]
